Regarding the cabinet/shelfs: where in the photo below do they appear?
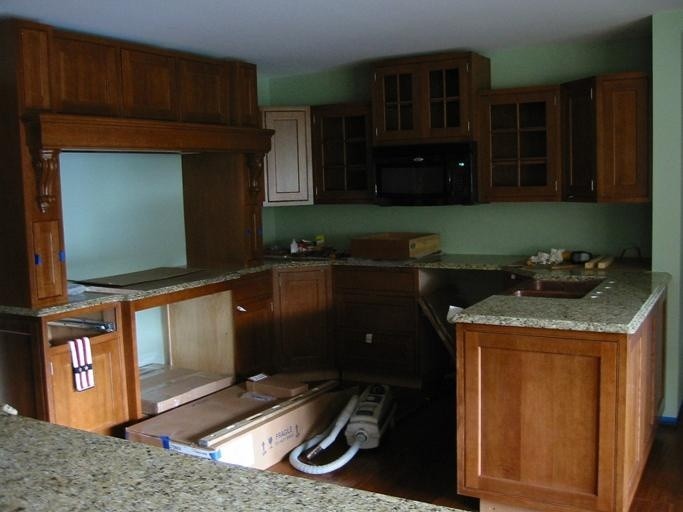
[276,264,418,370]
[371,52,469,140]
[480,73,651,203]
[263,107,316,207]
[316,103,367,204]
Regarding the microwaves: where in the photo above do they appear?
[375,142,478,204]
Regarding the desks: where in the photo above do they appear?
[1,414,465,512]
